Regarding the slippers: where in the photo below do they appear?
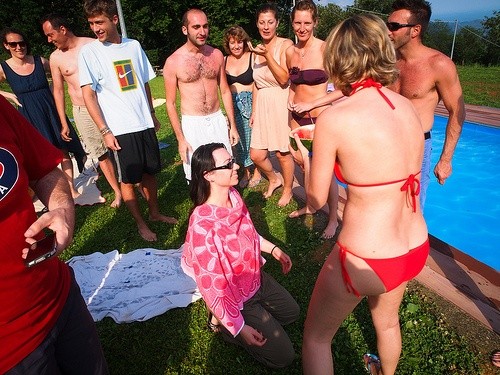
[363,353,380,375]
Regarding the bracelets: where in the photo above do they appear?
[271,245,278,255]
[151,110,155,113]
[101,126,111,135]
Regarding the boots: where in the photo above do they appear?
[207,311,221,332]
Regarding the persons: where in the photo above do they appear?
[163,8,240,185]
[181,143,301,368]
[285,0,345,239]
[387,0,465,216]
[302,14,429,375]
[249,4,294,207]
[41,0,180,241]
[0,28,87,198]
[223,25,262,189]
[491,351,500,367]
[0,94,112,375]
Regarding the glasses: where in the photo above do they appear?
[5,41,26,48]
[203,157,236,175]
[386,22,415,31]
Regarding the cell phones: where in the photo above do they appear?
[26,232,56,267]
